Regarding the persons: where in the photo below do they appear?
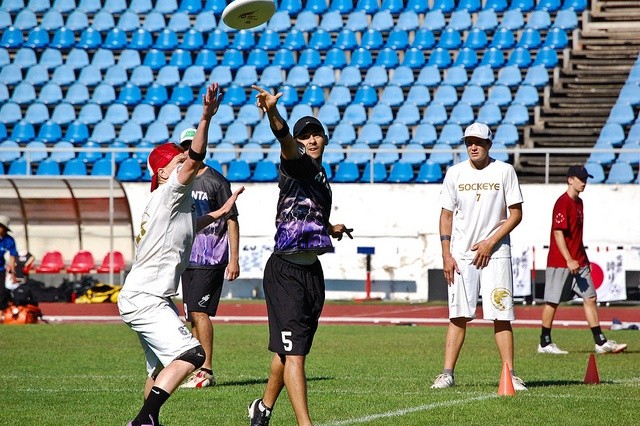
[180,128,239,389]
[429,123,527,390]
[247,85,353,426]
[0,216,16,310]
[537,165,627,355]
[117,82,245,426]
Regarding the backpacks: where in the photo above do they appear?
[75,283,120,303]
[12,284,37,304]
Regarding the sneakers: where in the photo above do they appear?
[178,370,216,389]
[511,375,527,391]
[538,343,569,354]
[430,373,454,389]
[247,397,272,425]
[595,340,627,354]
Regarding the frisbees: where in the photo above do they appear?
[222,0,276,28]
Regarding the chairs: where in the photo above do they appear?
[582,60,636,185]
[97,250,126,276]
[66,251,94,276]
[35,251,63,276]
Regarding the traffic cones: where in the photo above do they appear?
[583,350,600,385]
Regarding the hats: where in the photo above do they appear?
[460,122,493,142]
[0,216,12,231]
[292,116,324,138]
[147,142,184,192]
[180,127,197,146]
[566,164,594,180]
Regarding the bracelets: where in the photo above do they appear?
[441,235,450,241]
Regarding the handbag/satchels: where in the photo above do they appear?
[2,304,42,325]
